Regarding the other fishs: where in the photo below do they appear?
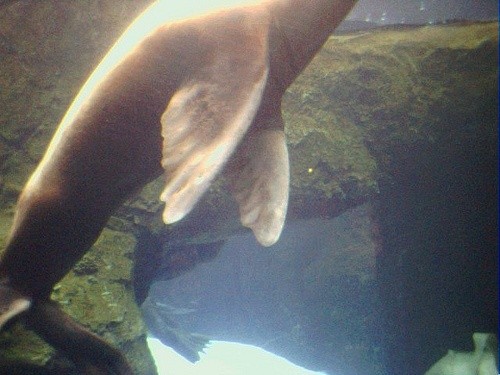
[0,0,359,375]
